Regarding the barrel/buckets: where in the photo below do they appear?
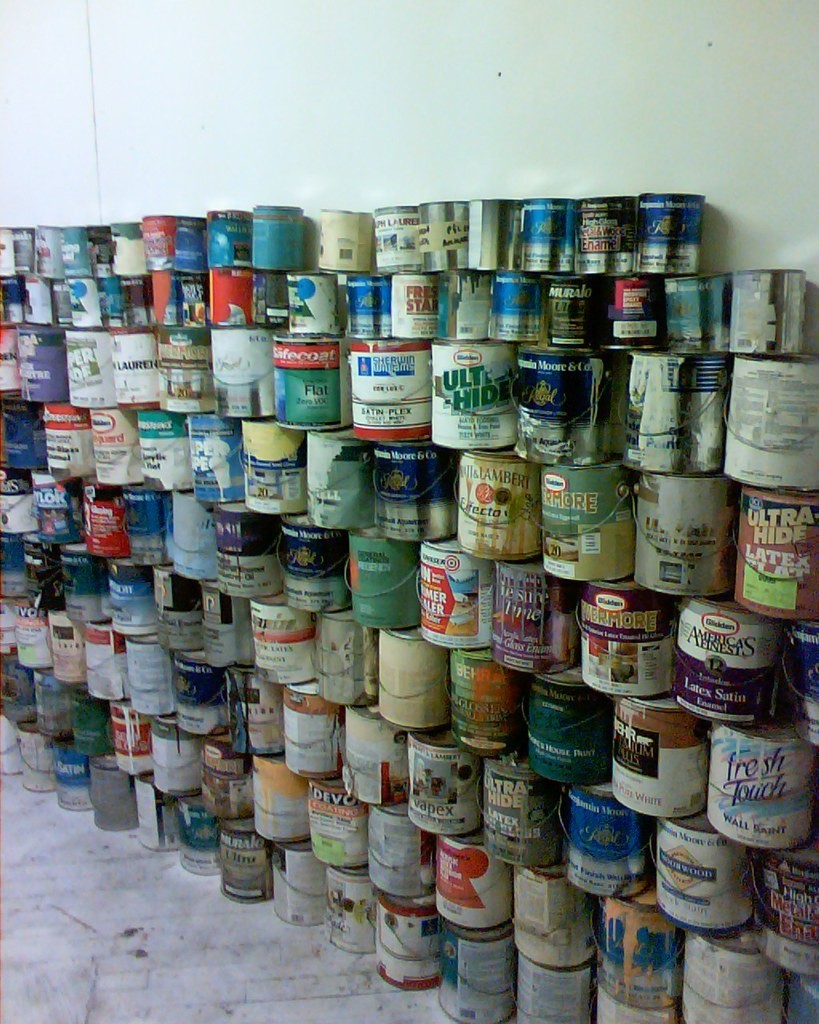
[1,191,819,1024]
